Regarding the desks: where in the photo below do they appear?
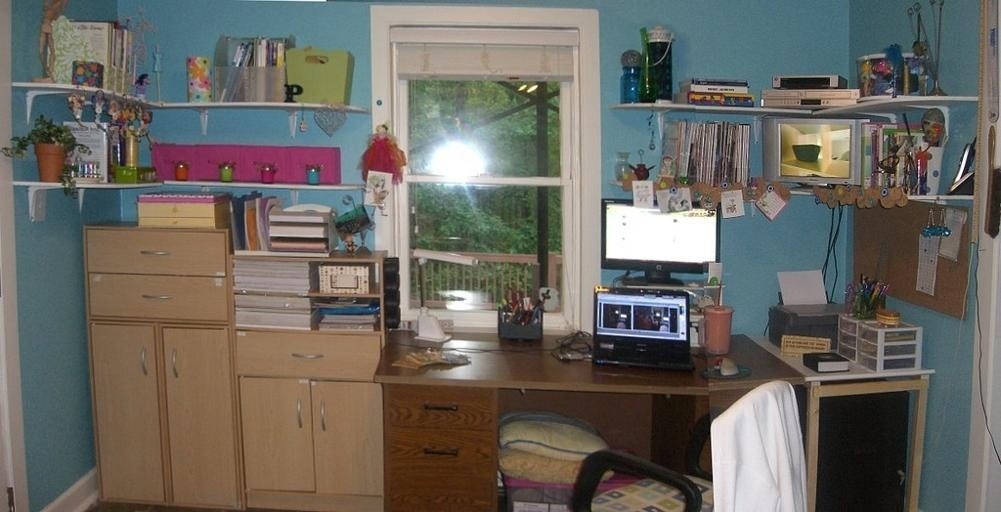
[749,335,936,512]
[371,328,805,510]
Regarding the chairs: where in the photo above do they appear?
[569,371,808,512]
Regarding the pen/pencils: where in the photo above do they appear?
[856,274,891,299]
[504,289,551,324]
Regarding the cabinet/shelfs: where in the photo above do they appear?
[13,82,372,224]
[81,225,246,512]
[608,93,979,202]
[229,250,390,511]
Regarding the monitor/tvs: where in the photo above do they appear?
[601,198,721,286]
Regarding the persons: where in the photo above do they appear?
[364,124,407,181]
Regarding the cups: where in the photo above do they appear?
[697,305,736,355]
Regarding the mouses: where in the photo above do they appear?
[719,357,739,376]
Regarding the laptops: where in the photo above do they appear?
[592,285,695,371]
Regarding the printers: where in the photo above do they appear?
[768,290,846,350]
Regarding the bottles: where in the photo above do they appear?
[638,26,657,103]
[619,48,641,103]
[643,23,675,102]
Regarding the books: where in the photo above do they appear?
[63,119,128,183]
[218,35,288,103]
[677,79,754,107]
[859,121,928,197]
[45,20,137,96]
[227,191,381,333]
[659,121,751,189]
[802,352,850,372]
